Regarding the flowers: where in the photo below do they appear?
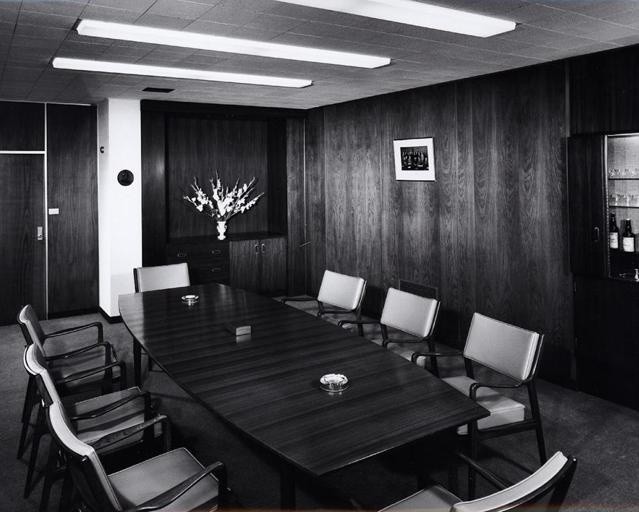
[183,170,266,219]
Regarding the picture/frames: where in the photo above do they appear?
[393,137,436,182]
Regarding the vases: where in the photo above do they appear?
[218,221,227,238]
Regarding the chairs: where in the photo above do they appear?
[279,267,367,335]
[132,262,192,293]
[337,285,441,377]
[411,313,548,501]
[377,450,579,512]
[19,303,234,510]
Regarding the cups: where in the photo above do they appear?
[609,166,639,176]
[611,192,633,207]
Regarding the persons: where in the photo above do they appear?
[401,151,428,170]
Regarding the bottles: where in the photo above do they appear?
[620,218,635,266]
[608,213,619,267]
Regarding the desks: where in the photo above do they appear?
[118,283,492,511]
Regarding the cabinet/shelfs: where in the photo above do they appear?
[142,100,291,302]
[0,100,46,327]
[603,134,639,282]
[45,105,100,318]
